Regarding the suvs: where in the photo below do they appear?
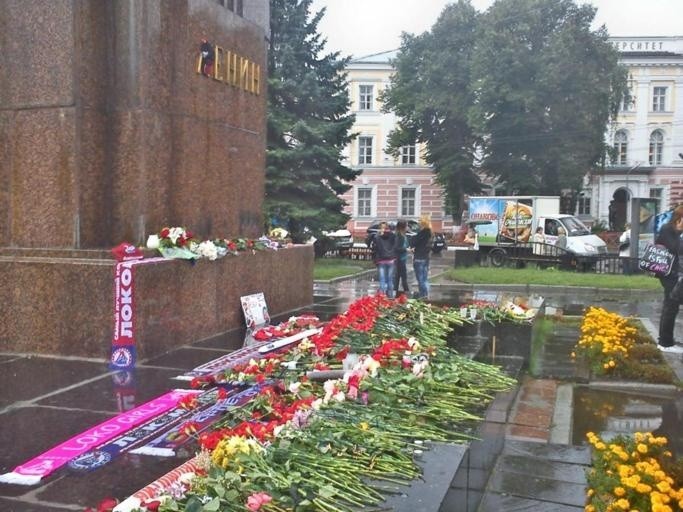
[365,217,447,255]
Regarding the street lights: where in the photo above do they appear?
[625,160,642,226]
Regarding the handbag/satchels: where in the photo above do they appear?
[617,229,630,252]
[638,241,676,276]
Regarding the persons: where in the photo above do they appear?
[546,221,554,235]
[467,222,478,249]
[396,219,410,293]
[655,205,683,353]
[411,216,432,298]
[554,227,566,256]
[533,228,545,259]
[372,222,398,298]
[620,223,631,273]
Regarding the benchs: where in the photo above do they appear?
[325,234,353,257]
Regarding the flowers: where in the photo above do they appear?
[93,290,521,512]
[147,222,292,258]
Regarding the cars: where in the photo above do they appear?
[618,210,683,272]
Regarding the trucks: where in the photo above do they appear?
[320,226,354,256]
[467,195,608,270]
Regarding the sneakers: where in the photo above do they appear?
[655,339,683,354]
[388,287,429,301]
